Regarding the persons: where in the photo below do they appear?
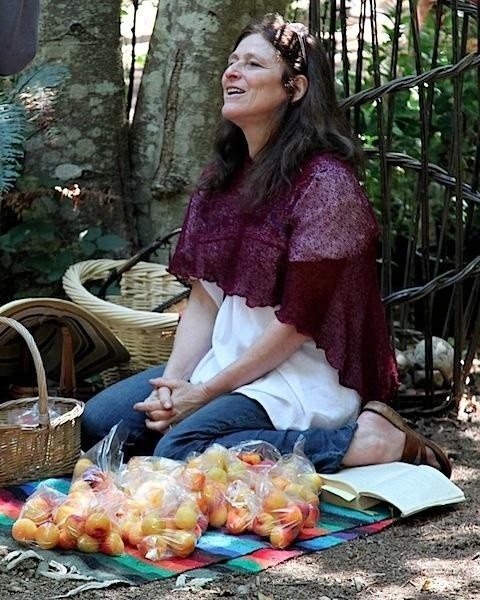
[81,12,451,478]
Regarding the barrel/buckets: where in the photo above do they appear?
[362,400,452,479]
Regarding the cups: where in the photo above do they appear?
[287,18,310,70]
